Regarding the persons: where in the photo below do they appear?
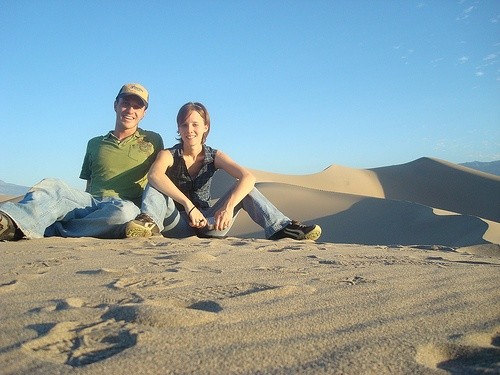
[126,101,322,240]
[0,83,164,240]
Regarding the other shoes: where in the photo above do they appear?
[0,211,17,240]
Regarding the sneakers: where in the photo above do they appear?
[269,220,321,240]
[125,213,164,237]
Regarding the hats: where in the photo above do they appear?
[116,83,149,108]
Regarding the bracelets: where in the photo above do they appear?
[189,206,196,215]
